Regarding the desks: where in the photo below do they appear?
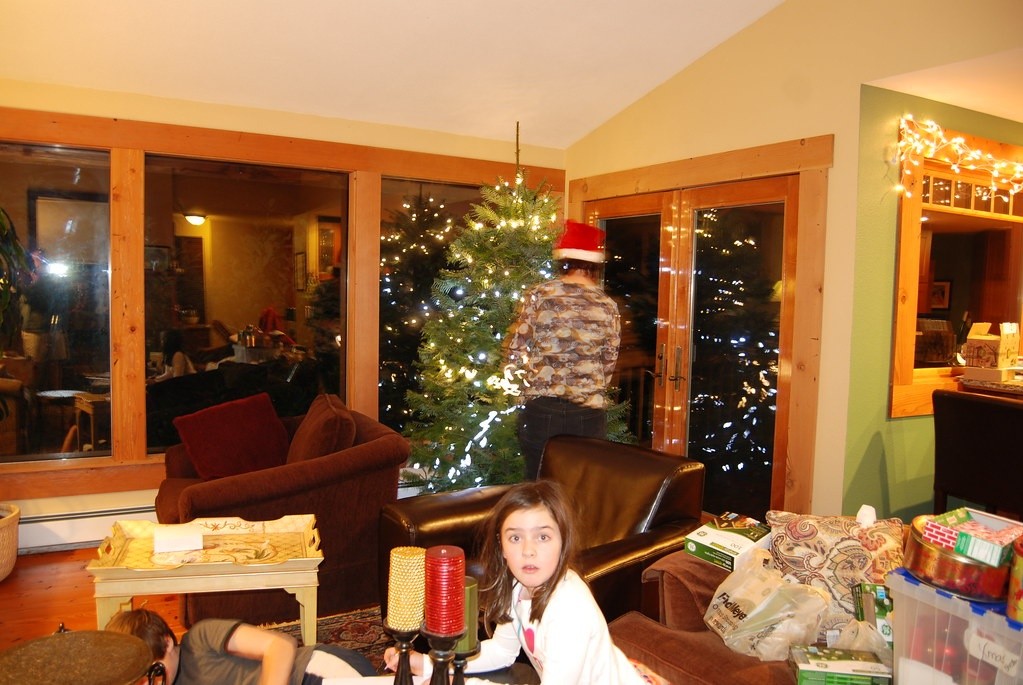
[89,514,334,649]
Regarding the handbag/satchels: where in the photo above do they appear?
[705,547,831,661]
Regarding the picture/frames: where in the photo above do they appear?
[929,280,949,308]
[293,252,307,292]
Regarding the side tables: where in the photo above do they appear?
[74,391,110,451]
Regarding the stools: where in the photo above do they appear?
[0,628,165,685]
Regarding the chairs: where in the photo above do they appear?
[930,388,1023,518]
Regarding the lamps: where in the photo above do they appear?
[185,212,203,225]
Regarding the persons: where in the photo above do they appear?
[500,219,620,481]
[384,481,649,685]
[105,609,378,685]
[170,305,233,378]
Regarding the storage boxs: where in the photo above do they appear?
[787,510,1023,685]
[964,321,1020,384]
[684,515,769,572]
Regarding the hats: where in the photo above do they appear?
[553,218,606,263]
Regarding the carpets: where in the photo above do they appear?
[258,606,399,675]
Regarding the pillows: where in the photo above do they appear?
[175,392,287,482]
[765,506,903,644]
[287,390,356,468]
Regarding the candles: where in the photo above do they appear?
[425,544,465,633]
[453,576,478,652]
[387,546,426,630]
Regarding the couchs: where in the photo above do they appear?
[378,433,718,642]
[157,403,414,637]
